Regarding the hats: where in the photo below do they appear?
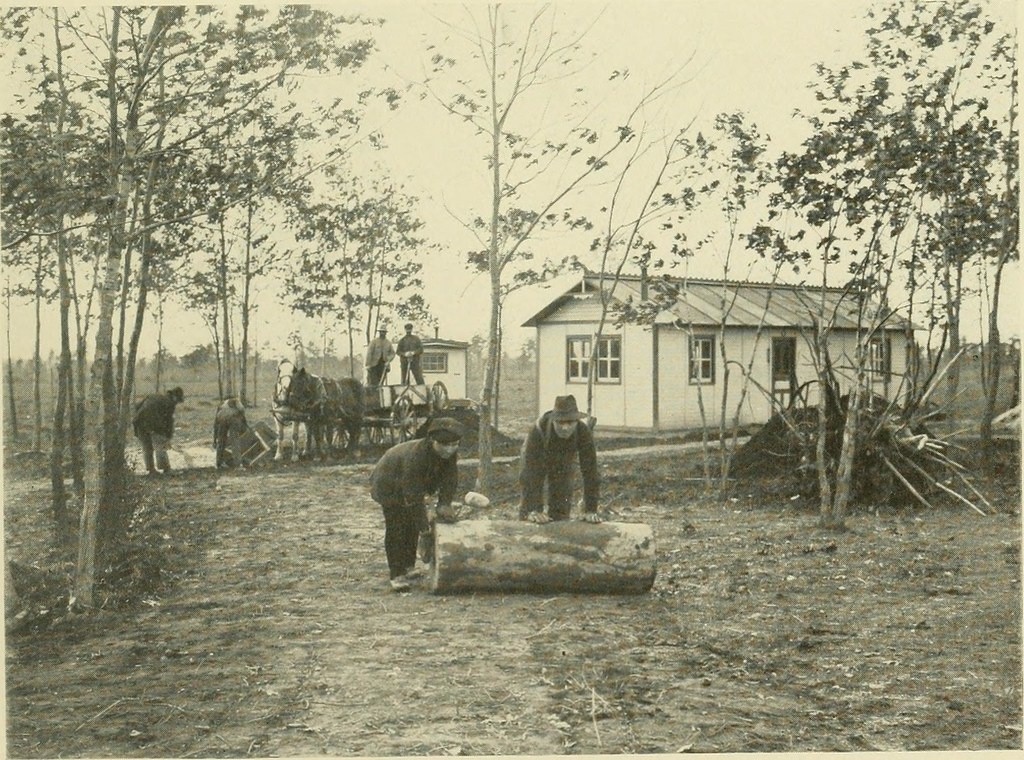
[166,386,184,402]
[544,395,589,421]
[405,323,414,329]
[426,417,464,436]
[376,324,389,332]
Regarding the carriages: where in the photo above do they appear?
[270,363,450,463]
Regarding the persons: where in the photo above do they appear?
[399,326,425,387]
[369,418,463,591]
[367,326,395,390]
[210,396,249,467]
[131,385,185,474]
[515,393,601,526]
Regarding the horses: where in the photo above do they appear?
[288,366,366,465]
[273,353,339,462]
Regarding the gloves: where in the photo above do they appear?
[434,504,458,524]
[417,531,433,564]
[578,512,603,524]
[528,510,553,524]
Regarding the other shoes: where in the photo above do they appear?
[390,566,422,593]
